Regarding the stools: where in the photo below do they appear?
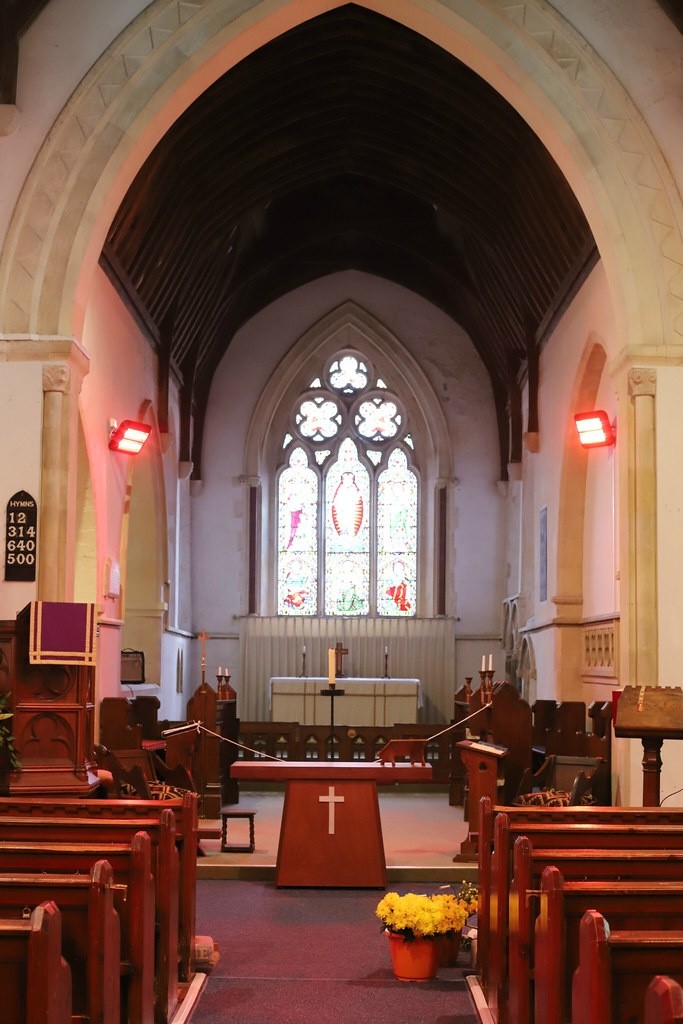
[219,809,258,852]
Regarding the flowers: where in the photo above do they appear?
[375,880,480,943]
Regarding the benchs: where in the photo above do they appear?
[512,755,611,807]
[0,791,199,1024]
[103,696,194,751]
[95,744,208,857]
[531,700,613,757]
[464,795,682,1024]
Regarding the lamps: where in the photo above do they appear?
[108,418,152,455]
[574,410,616,448]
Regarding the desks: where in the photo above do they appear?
[230,760,433,889]
[268,677,425,744]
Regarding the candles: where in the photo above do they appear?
[218,666,222,675]
[488,654,492,671]
[481,655,486,671]
[225,668,228,676]
[328,648,336,684]
[385,646,388,654]
[303,646,306,653]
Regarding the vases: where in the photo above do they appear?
[433,928,463,965]
[383,929,439,982]
